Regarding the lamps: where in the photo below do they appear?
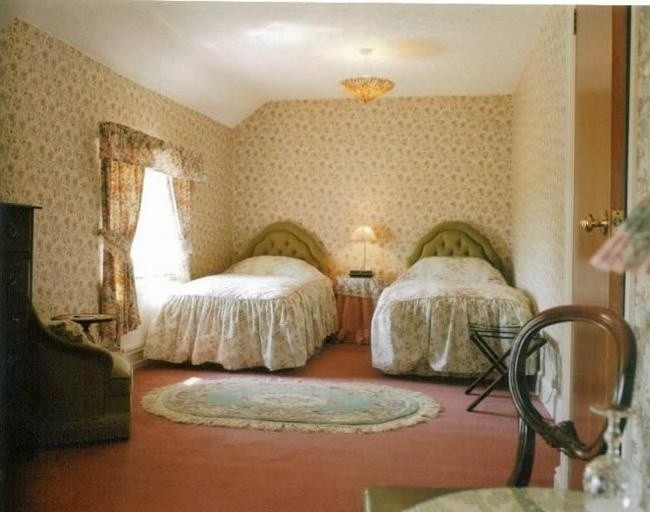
[349,224,377,278]
[337,49,396,103]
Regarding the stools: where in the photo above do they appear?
[465,323,546,411]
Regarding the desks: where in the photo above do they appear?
[50,312,115,347]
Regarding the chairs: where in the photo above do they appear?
[22,302,133,447]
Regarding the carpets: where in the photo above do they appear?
[141,372,440,432]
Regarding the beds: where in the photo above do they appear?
[373,218,538,385]
[146,219,333,371]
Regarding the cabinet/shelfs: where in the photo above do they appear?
[0,200,43,432]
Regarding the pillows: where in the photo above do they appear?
[47,320,89,346]
